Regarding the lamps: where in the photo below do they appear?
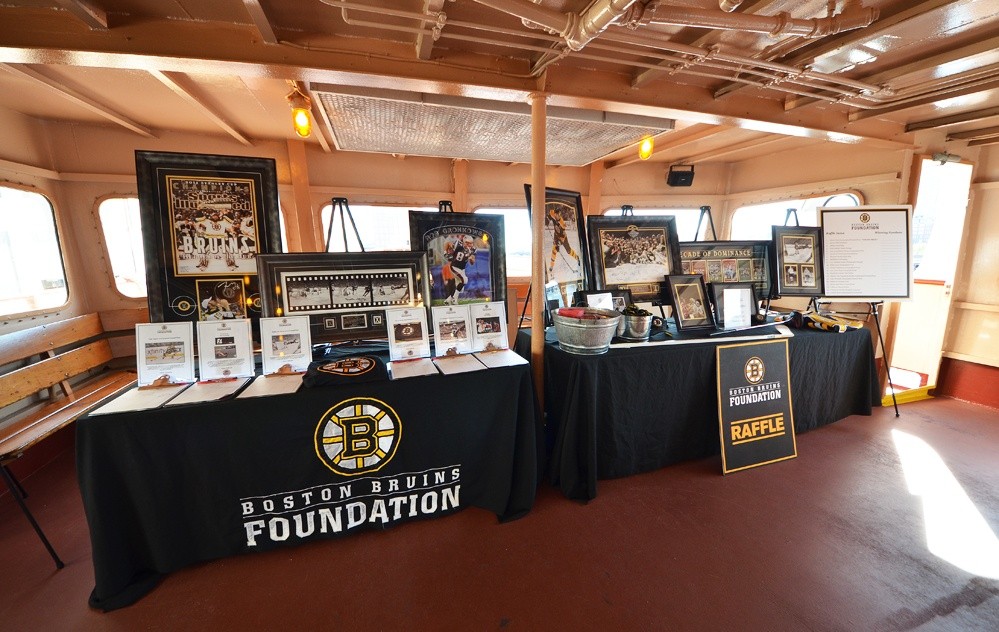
[285,85,312,138]
[666,164,695,186]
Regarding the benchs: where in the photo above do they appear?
[0,314,138,569]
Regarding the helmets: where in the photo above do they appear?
[463,235,474,243]
[554,208,561,213]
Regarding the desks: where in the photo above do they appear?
[72,334,539,614]
[518,313,881,504]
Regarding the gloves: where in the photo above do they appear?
[556,245,560,252]
[545,220,549,226]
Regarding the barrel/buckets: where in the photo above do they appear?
[551,307,623,355]
[616,313,652,343]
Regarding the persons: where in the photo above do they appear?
[601,231,666,267]
[441,234,476,305]
[202,297,236,320]
[546,208,580,271]
[172,181,257,269]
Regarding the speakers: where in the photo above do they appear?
[666,164,695,187]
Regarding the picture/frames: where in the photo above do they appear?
[134,149,508,377]
[523,182,825,332]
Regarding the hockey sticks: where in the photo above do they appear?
[547,226,579,274]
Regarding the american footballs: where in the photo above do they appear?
[443,240,454,255]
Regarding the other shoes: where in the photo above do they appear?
[549,268,552,273]
[578,260,580,266]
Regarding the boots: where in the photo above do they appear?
[444,295,453,306]
[453,290,460,305]
